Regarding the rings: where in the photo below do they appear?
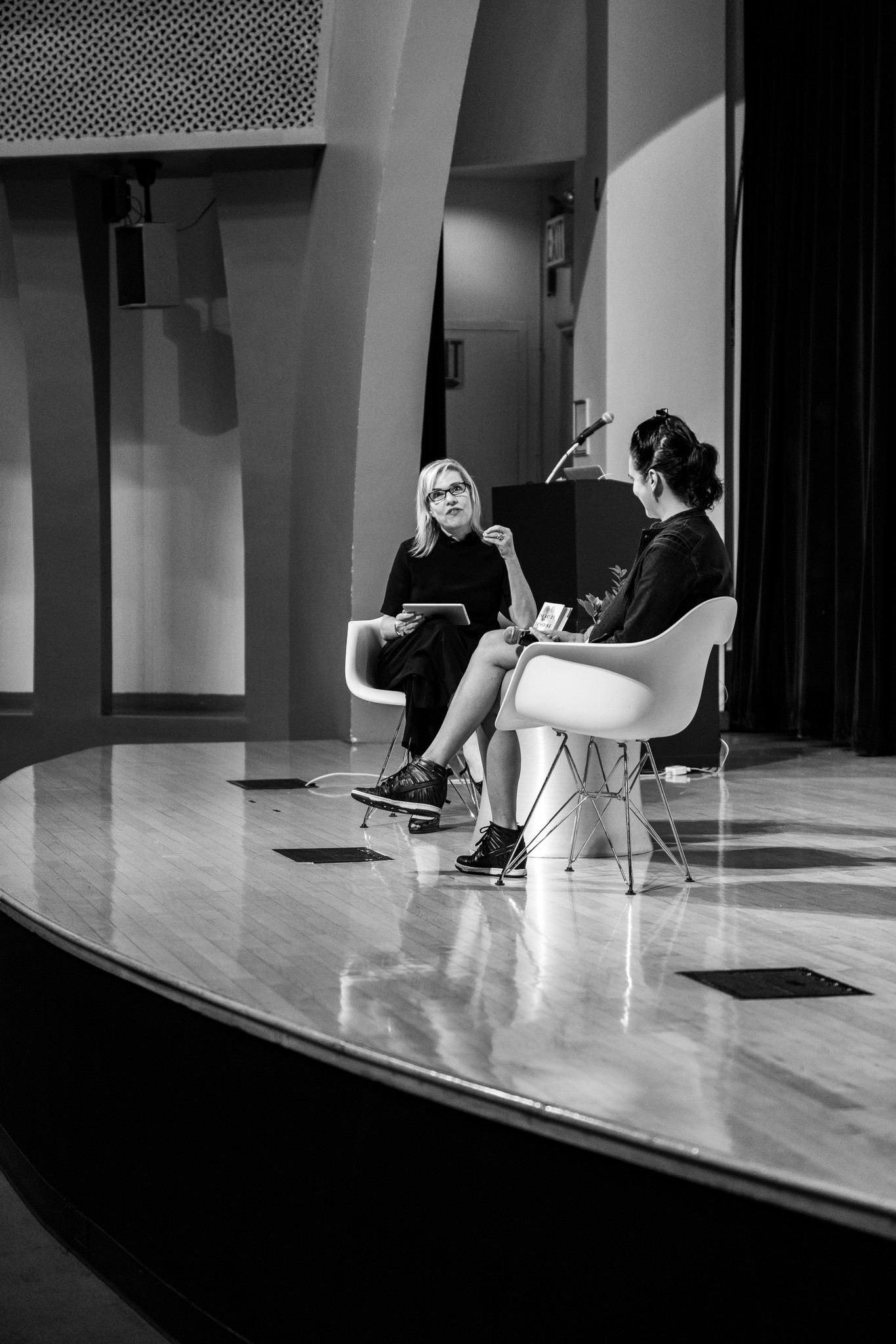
[497,532,504,540]
[401,621,407,626]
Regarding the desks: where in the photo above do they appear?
[471,726,652,858]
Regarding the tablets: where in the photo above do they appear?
[402,603,470,626]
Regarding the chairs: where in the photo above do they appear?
[346,616,480,827]
[493,597,738,894]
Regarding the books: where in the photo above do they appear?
[531,602,567,633]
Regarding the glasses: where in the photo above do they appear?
[427,483,470,502]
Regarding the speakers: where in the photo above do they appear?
[109,223,182,309]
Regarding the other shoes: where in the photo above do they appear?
[408,817,440,832]
[461,760,483,795]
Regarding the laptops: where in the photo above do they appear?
[563,464,608,480]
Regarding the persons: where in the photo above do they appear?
[369,459,540,833]
[350,407,734,877]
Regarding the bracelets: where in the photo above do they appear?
[394,621,403,636]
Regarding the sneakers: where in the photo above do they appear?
[455,820,527,877]
[351,755,454,818]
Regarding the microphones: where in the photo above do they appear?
[503,626,540,647]
[575,412,614,443]
